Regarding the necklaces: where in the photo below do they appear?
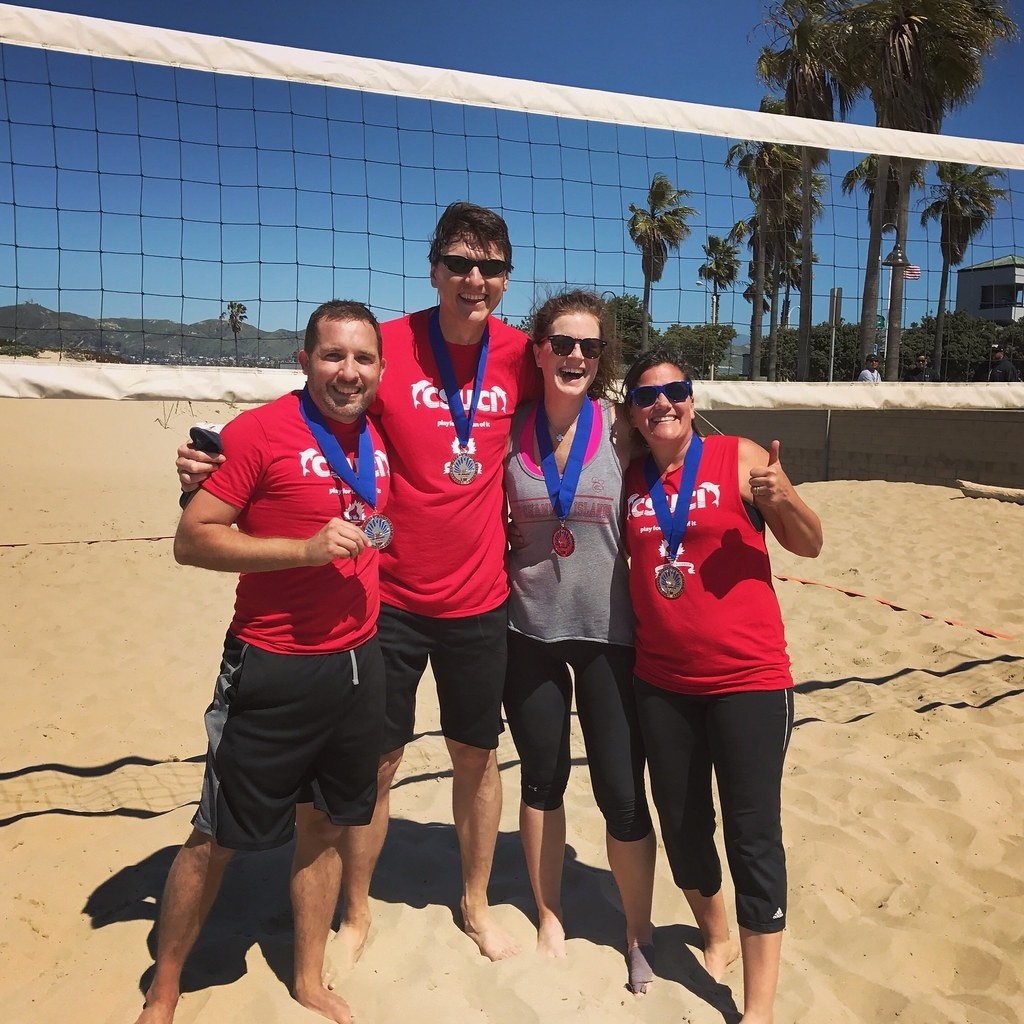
[546,414,581,441]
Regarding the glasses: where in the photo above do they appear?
[439,255,511,277]
[917,360,925,363]
[538,335,607,359]
[629,381,691,407]
[870,360,878,362]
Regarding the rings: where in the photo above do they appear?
[755,487,759,496]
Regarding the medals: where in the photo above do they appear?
[361,513,393,549]
[656,564,686,599]
[552,525,575,557]
[449,453,477,486]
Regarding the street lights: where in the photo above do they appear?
[697,280,716,381]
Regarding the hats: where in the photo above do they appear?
[866,354,877,360]
[992,344,1005,352]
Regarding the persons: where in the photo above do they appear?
[902,355,940,382]
[175,200,544,991]
[508,345,824,1024]
[501,289,658,996]
[132,299,393,1023]
[973,344,1020,382]
[857,354,882,382]
